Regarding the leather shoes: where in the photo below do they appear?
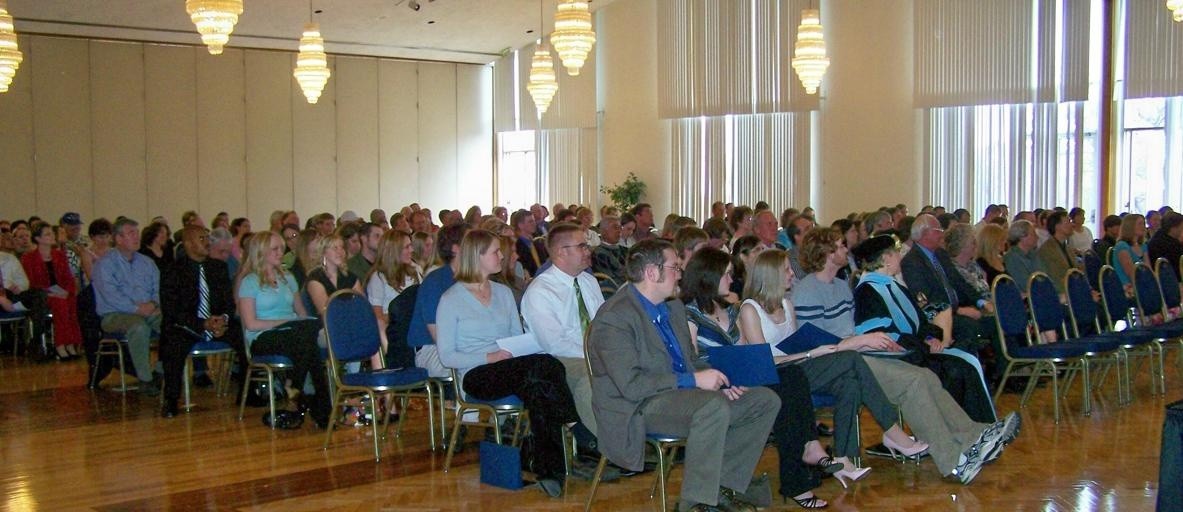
[234,390,267,407]
[159,390,179,417]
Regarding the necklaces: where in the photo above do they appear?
[471,281,495,303]
[709,302,724,326]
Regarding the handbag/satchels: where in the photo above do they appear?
[477,440,523,491]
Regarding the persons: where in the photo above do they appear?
[1,201,1183,512]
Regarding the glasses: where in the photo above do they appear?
[655,262,681,273]
[932,225,945,237]
[560,241,588,252]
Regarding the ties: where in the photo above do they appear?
[931,256,961,307]
[572,277,591,338]
[0,269,7,299]
[197,262,211,322]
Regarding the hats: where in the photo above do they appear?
[58,212,83,226]
[858,237,897,262]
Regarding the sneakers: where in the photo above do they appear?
[528,456,562,496]
[191,372,213,390]
[306,400,335,432]
[983,411,1022,466]
[575,438,622,467]
[956,436,999,489]
[138,381,157,400]
[261,409,308,431]
[439,420,468,452]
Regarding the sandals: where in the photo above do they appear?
[818,422,832,438]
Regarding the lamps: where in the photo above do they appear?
[0,0,22,92]
[185,0,243,55]
[792,0,830,96]
[293,0,331,104]
[525,0,558,113]
[548,0,596,77]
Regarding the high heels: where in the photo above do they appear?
[880,436,929,460]
[778,489,828,509]
[379,397,400,423]
[831,464,875,491]
[67,346,80,361]
[354,405,373,426]
[52,348,69,361]
[797,452,843,476]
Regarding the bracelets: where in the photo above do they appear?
[219,313,229,332]
[806,349,811,360]
